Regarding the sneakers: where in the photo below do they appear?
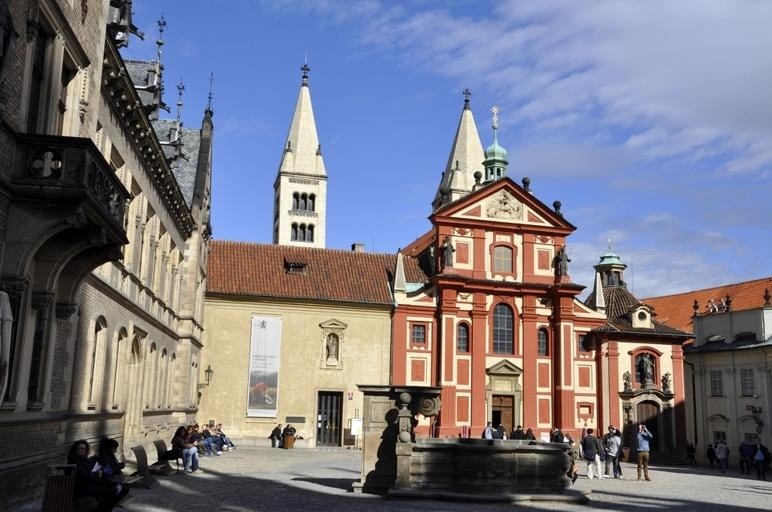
[192,468,204,474]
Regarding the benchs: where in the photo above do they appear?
[167,449,183,474]
[149,463,167,471]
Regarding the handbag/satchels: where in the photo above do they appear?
[584,450,595,461]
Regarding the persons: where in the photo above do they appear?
[602,428,623,479]
[634,423,652,481]
[483,421,564,442]
[581,428,605,479]
[278,424,296,447]
[251,380,271,404]
[96,437,129,508]
[268,424,282,448]
[602,425,624,478]
[688,437,770,481]
[327,334,337,358]
[556,247,570,275]
[67,439,122,511]
[171,423,238,474]
[501,193,510,206]
[639,353,656,381]
[440,235,455,265]
